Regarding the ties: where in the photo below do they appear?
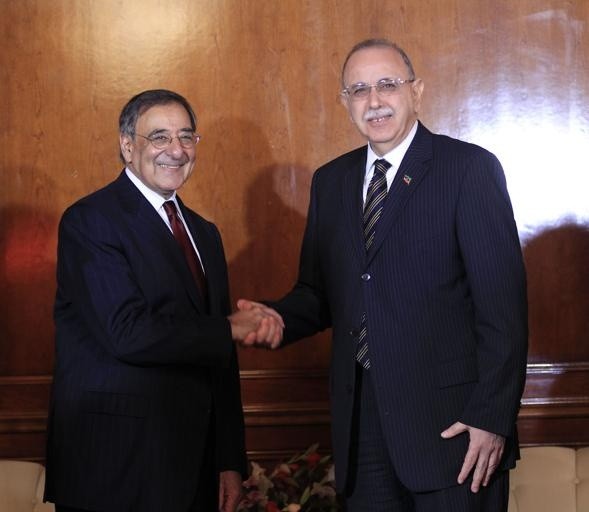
[162,200,207,303]
[354,158,393,374]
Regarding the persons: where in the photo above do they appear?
[236,36,529,511]
[43,90,269,510]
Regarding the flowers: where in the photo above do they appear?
[240,442,338,512]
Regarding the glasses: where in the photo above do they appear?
[341,76,420,97]
[134,130,201,150]
